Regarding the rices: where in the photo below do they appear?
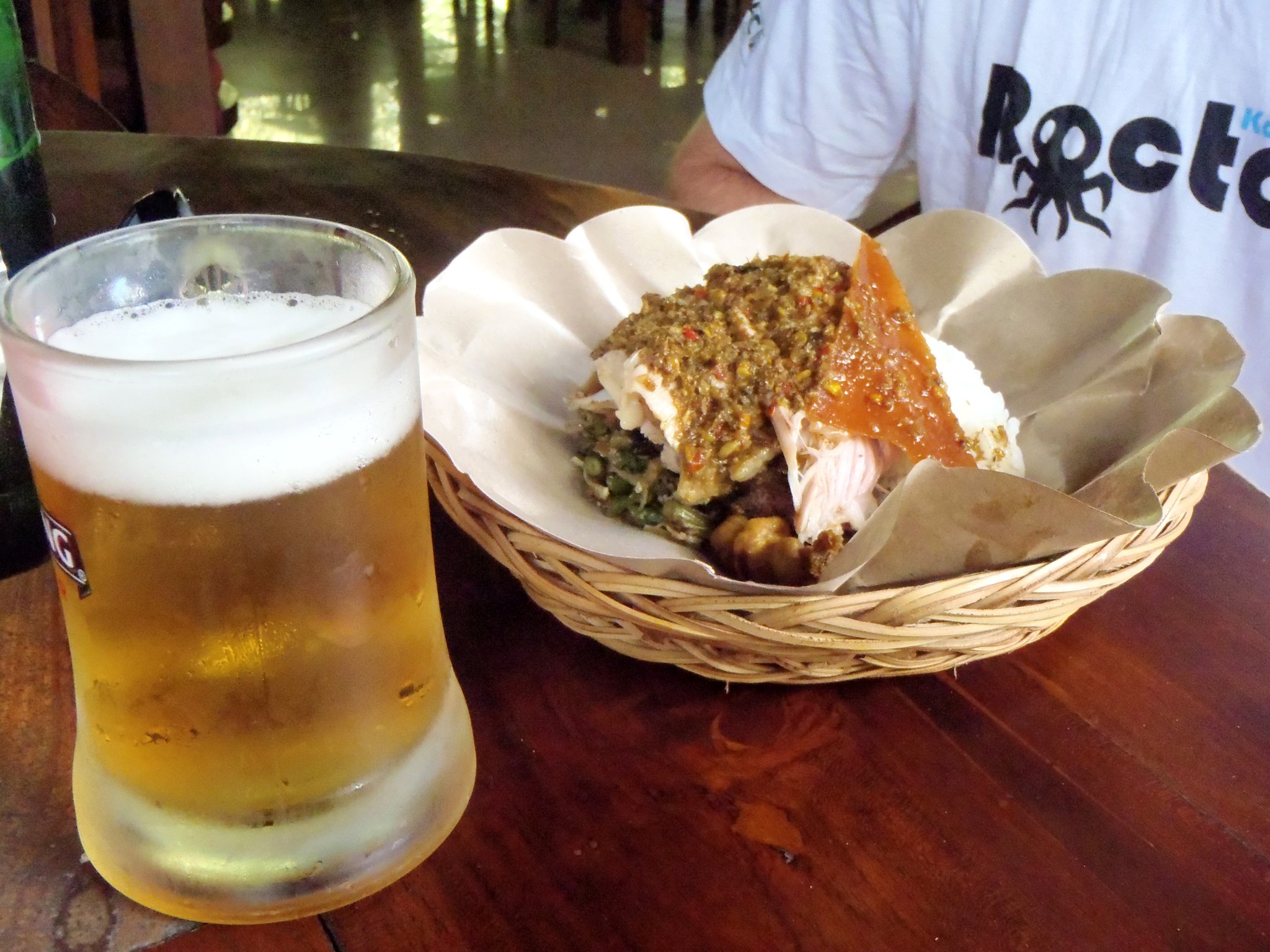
[918,328,1027,480]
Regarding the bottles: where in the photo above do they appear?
[0,0,61,579]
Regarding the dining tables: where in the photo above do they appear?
[1,128,1270,952]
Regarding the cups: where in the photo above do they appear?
[2,213,476,924]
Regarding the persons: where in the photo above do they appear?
[672,1,1270,497]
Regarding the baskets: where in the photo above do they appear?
[423,426,1211,689]
